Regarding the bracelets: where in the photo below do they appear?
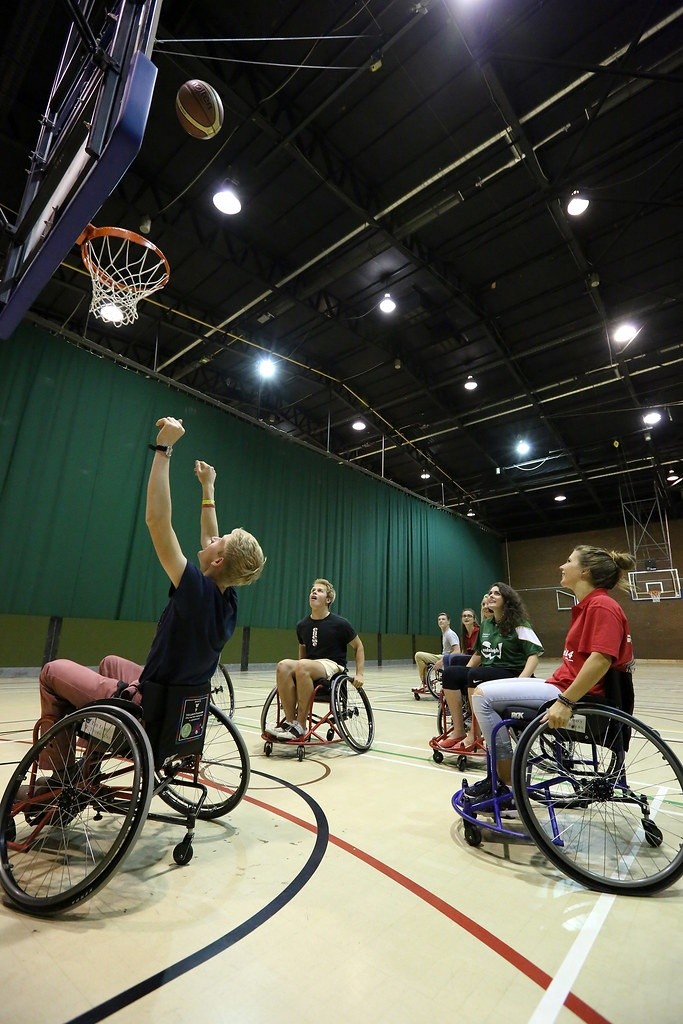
[201,499,215,508]
[557,693,578,711]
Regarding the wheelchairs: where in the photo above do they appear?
[260,672,376,762]
[412,662,535,771]
[451,667,683,897]
[1,698,251,916]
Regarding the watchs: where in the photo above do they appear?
[147,443,174,457]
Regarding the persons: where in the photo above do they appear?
[12,415,263,801]
[263,577,365,741]
[410,593,493,723]
[438,581,545,754]
[460,546,637,822]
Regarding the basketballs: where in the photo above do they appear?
[174,80,224,139]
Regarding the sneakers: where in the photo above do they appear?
[278,723,305,740]
[264,720,292,738]
[464,771,504,798]
[471,783,520,818]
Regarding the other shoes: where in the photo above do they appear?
[16,784,53,805]
[440,734,467,748]
[413,686,429,692]
[451,740,483,751]
[37,777,61,786]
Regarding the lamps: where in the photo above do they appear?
[352,416,366,430]
[591,272,600,287]
[567,189,590,215]
[380,278,396,314]
[464,375,478,390]
[139,214,151,234]
[212,175,242,215]
